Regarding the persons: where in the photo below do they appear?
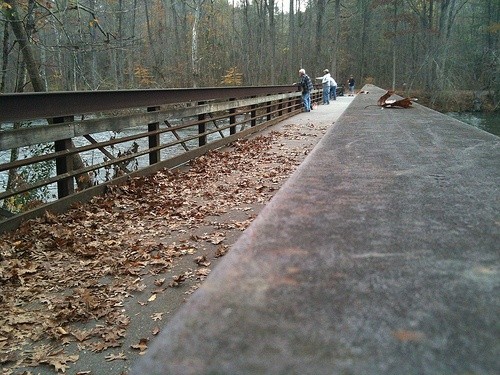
[330,76,338,100]
[292,68,313,112]
[346,73,354,96]
[321,68,331,105]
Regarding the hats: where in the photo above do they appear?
[299,69,305,74]
[323,69,329,72]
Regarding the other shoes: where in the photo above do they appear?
[302,110,310,112]
